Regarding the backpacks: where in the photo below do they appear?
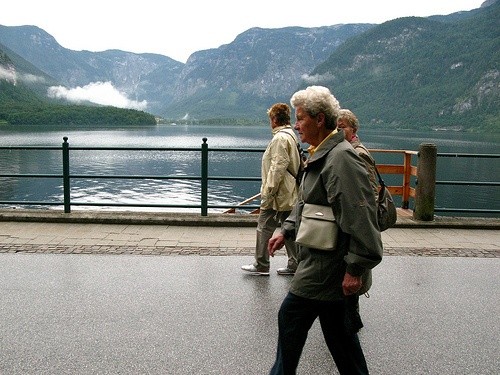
[278,131,307,186]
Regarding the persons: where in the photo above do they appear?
[336,108,398,230]
[267,85,383,375]
[242,103,307,276]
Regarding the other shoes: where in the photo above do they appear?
[276,266,296,274]
[241,264,270,276]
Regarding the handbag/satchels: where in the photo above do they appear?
[373,163,397,233]
[294,202,340,251]
